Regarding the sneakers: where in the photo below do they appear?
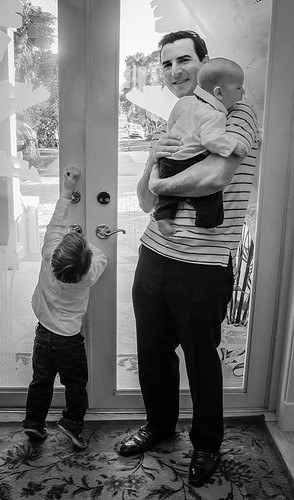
[23,422,88,450]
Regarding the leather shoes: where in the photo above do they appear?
[118,423,222,488]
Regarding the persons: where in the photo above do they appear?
[23,170,108,449]
[154,58,247,237]
[120,30,260,482]
